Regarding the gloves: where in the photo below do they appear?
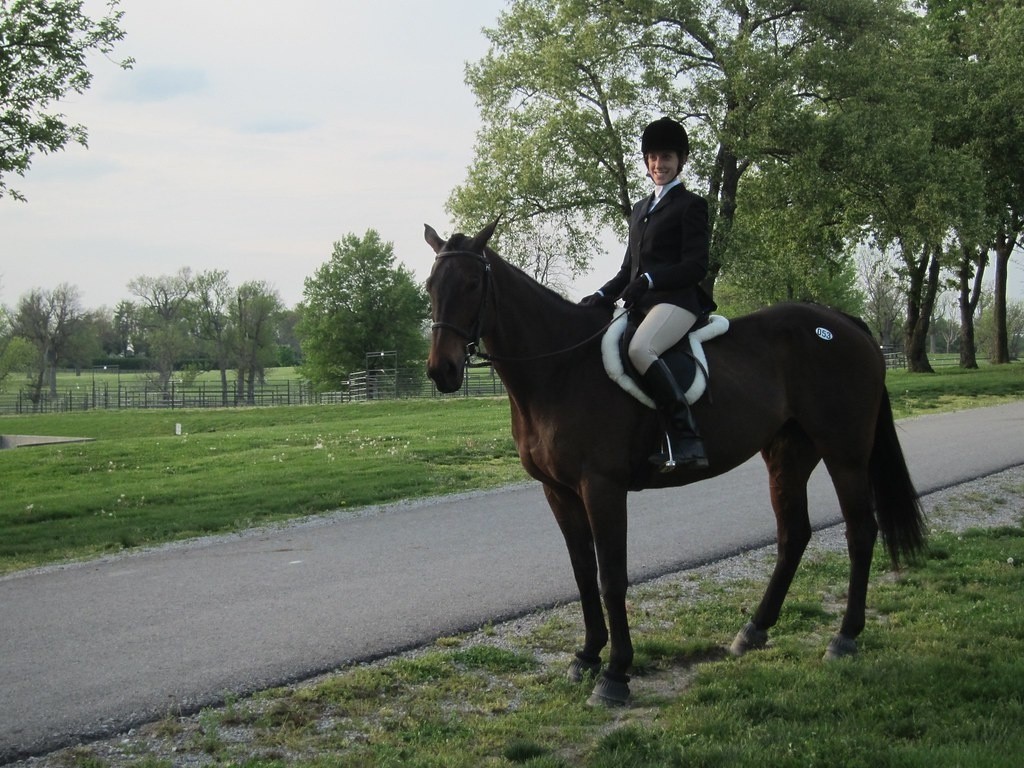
[578,292,602,305]
[618,274,649,310]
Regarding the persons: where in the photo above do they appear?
[581,117,709,469]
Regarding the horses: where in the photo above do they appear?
[422,214,925,707]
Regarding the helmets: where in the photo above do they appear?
[642,117,689,151]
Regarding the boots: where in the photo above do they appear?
[640,359,709,469]
